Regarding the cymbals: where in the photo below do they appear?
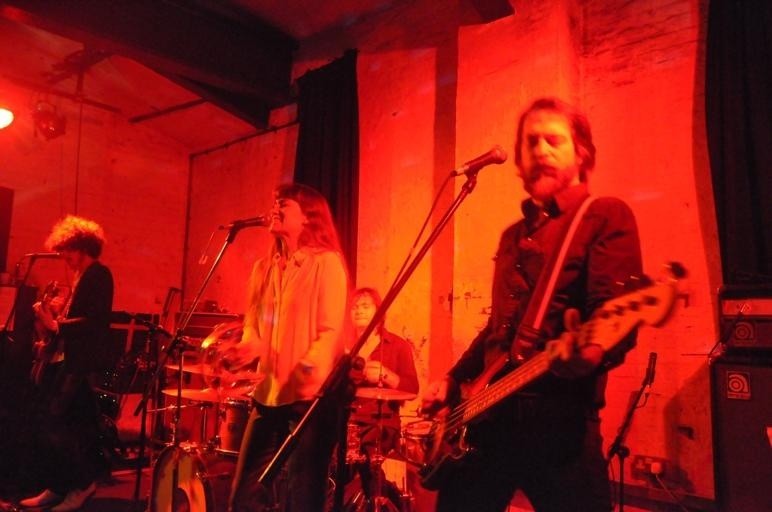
[162,384,253,405]
[354,386,417,401]
[162,362,266,381]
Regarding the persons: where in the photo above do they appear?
[418,94,643,512]
[235,183,345,512]
[20,215,117,511]
[345,288,419,400]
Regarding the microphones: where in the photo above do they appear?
[645,352,656,397]
[26,250,64,259]
[451,145,508,177]
[220,214,271,229]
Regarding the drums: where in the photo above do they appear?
[403,418,433,466]
[214,397,253,457]
[150,440,237,512]
[332,424,360,466]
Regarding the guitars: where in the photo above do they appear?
[30,280,63,385]
[418,260,685,490]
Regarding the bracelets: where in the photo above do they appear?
[297,359,317,377]
[382,370,389,381]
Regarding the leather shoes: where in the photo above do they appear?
[51,482,99,512]
[20,489,63,507]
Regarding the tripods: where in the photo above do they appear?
[351,401,399,512]
